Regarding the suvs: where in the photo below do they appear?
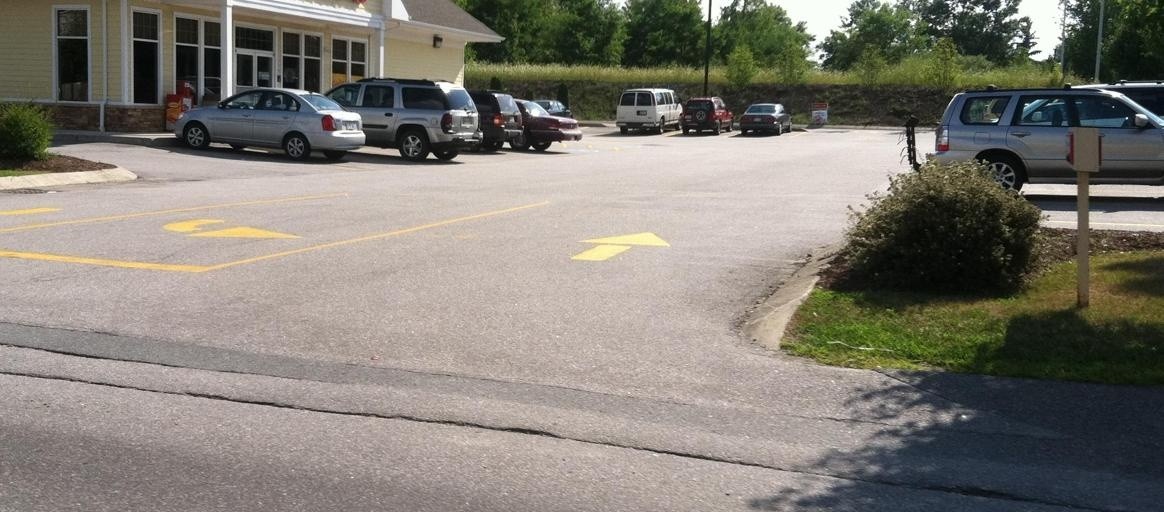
[926,84,1164,200]
[320,76,483,163]
[680,96,734,136]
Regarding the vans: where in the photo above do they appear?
[991,79,1164,129]
[460,90,524,154]
[615,88,683,136]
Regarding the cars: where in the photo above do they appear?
[174,76,266,103]
[508,99,581,153]
[739,103,792,136]
[174,89,366,161]
[532,100,572,119]
